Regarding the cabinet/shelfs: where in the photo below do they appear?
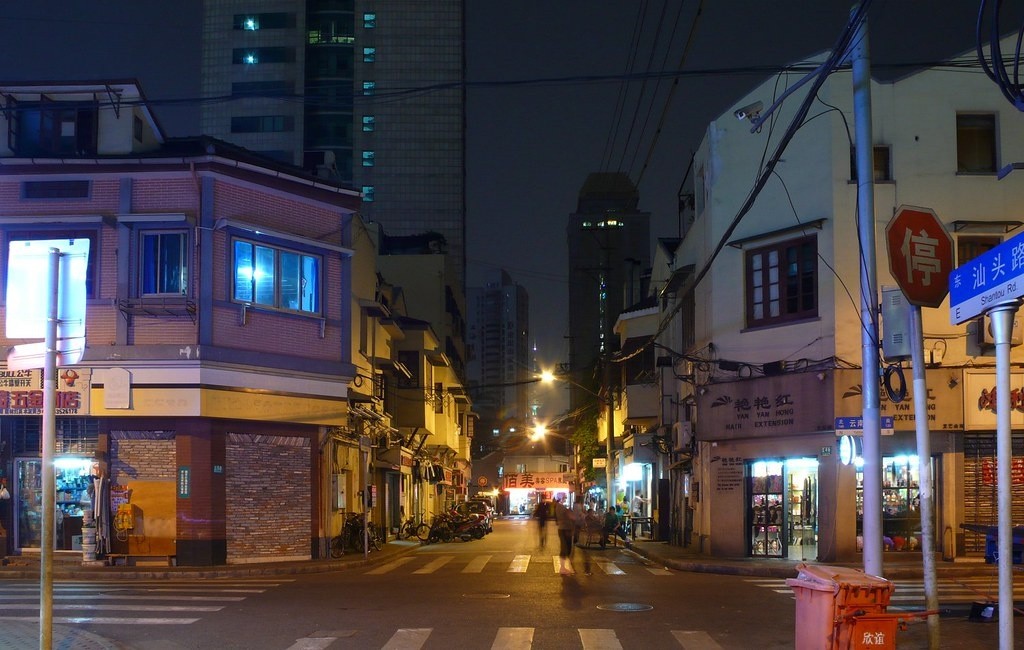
[751,489,815,556]
[54,487,92,549]
[856,486,921,551]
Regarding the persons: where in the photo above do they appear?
[605,506,632,543]
[616,505,623,516]
[776,505,782,525]
[589,498,595,511]
[574,495,585,546]
[0,473,14,556]
[586,509,611,544]
[770,540,777,549]
[760,505,765,524]
[753,505,760,524]
[890,490,897,501]
[885,490,890,501]
[768,506,776,524]
[533,493,551,548]
[634,490,650,538]
[758,540,764,551]
[620,496,628,512]
[554,492,577,575]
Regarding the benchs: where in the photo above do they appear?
[104,552,176,567]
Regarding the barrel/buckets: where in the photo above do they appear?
[80,509,97,562]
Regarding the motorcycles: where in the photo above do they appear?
[441,509,485,541]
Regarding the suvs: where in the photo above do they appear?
[468,494,494,514]
[461,501,494,534]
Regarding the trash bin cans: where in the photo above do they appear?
[784,563,895,650]
[986,525,1024,566]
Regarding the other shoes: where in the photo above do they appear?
[599,541,606,547]
[624,536,630,542]
[560,569,570,574]
[606,539,611,543]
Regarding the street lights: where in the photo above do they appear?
[534,426,580,501]
[542,371,613,512]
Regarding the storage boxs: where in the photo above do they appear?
[469,514,479,521]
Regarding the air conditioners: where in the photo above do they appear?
[671,421,691,451]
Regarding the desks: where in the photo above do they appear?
[631,517,654,541]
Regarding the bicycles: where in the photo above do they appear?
[330,511,382,559]
[401,508,431,541]
[427,511,454,545]
